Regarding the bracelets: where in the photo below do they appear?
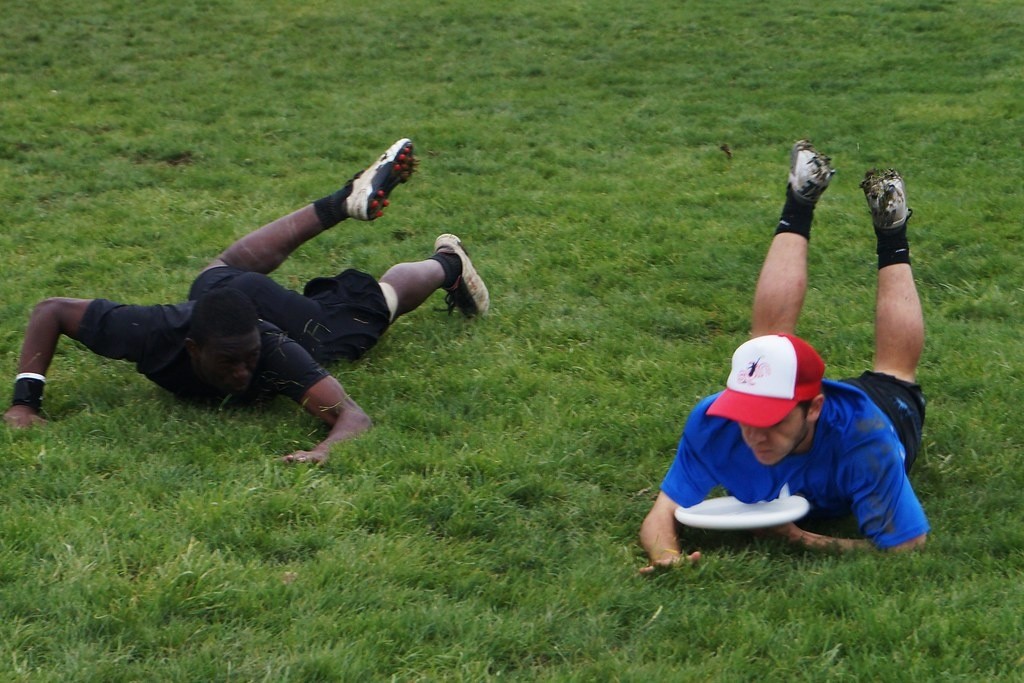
[13,371,47,412]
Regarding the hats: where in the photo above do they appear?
[705,332,825,428]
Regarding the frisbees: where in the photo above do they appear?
[674,495,810,530]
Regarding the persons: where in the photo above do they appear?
[5,138,488,467]
[639,139,932,574]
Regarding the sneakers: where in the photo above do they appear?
[343,137,414,222]
[434,234,490,318]
[787,138,832,203]
[864,166,914,230]
